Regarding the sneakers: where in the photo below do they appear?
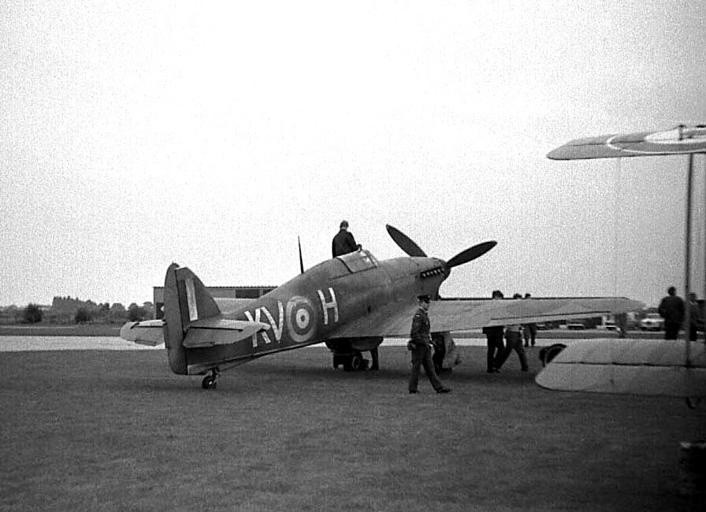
[435,386,451,394]
[409,390,418,393]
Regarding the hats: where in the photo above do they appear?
[416,294,431,303]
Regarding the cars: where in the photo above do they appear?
[605,313,635,330]
[536,322,553,330]
[567,319,593,330]
[640,312,664,331]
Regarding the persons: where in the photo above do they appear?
[493,291,531,373]
[331,220,357,258]
[658,286,686,340]
[480,290,507,373]
[685,290,703,339]
[409,292,454,396]
[524,293,538,348]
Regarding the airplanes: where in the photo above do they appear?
[120,224,646,389]
